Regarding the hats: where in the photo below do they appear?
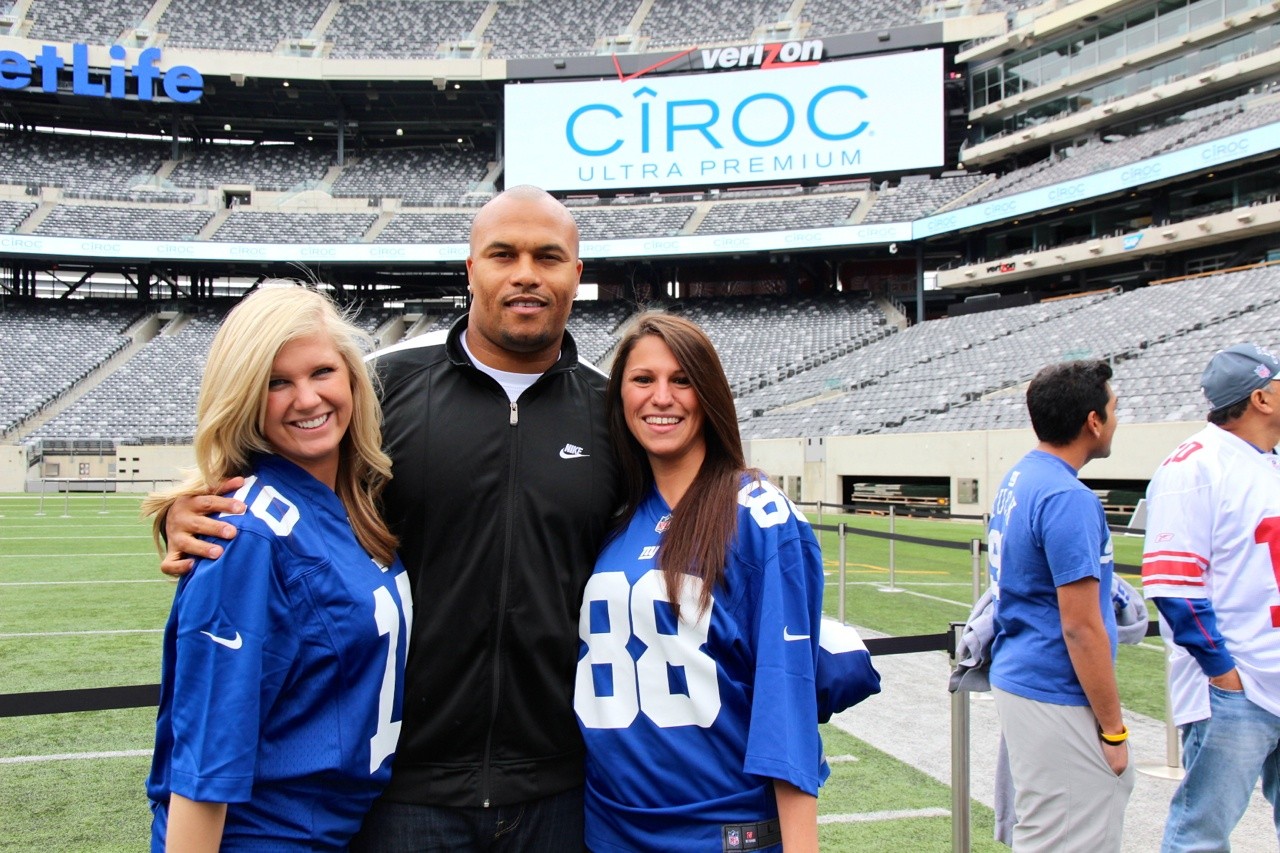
[1200,344,1280,412]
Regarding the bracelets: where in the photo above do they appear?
[1098,725,1129,746]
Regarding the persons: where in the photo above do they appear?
[161,188,624,853]
[143,287,414,853]
[1141,345,1280,853]
[574,315,831,853]
[988,359,1135,853]
